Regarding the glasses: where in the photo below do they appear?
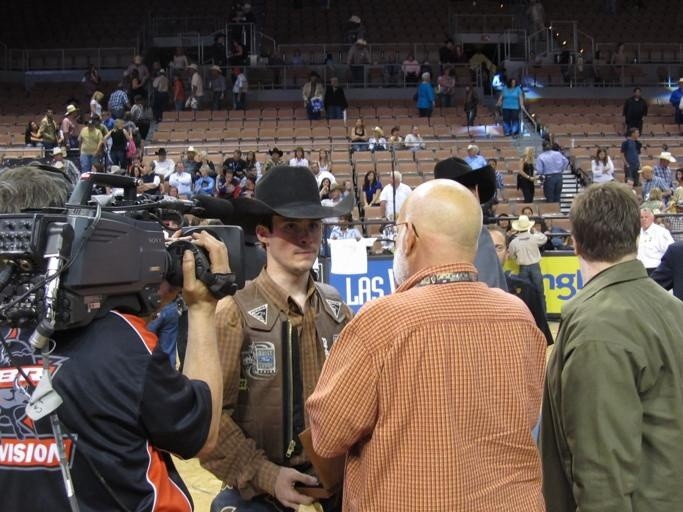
[382,223,420,239]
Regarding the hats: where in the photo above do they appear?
[209,65,222,73]
[49,146,65,156]
[188,145,197,154]
[310,71,320,78]
[676,77,683,83]
[638,164,653,173]
[160,69,165,73]
[356,39,367,46]
[233,165,355,220]
[372,125,384,137]
[84,119,96,125]
[512,214,534,231]
[65,105,79,116]
[270,147,283,157]
[109,165,125,174]
[435,157,496,203]
[186,63,198,71]
[133,56,141,64]
[652,151,676,162]
[154,148,167,154]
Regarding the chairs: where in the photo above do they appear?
[518,95,683,223]
[2,82,138,163]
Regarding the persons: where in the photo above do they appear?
[198,163,354,512]
[25,0,683,347]
[536,180,683,512]
[1,164,230,512]
[303,177,550,512]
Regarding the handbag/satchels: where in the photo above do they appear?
[211,489,287,511]
[413,94,418,100]
[126,139,136,159]
[185,96,198,109]
